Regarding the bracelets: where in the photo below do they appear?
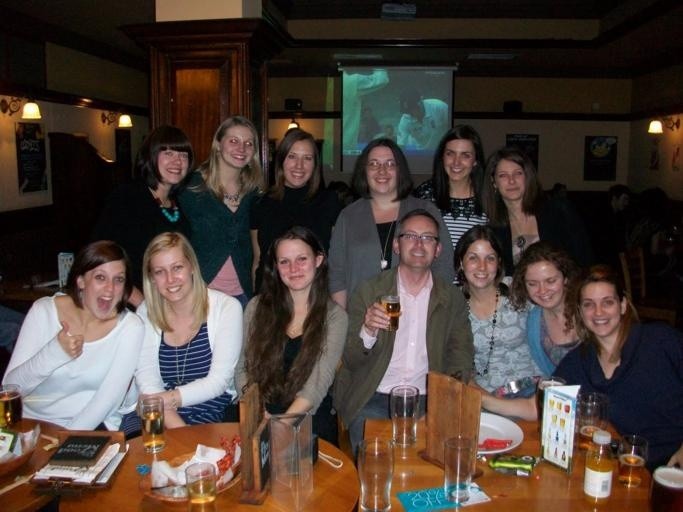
[168,388,178,412]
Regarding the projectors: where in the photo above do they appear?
[380,3,417,22]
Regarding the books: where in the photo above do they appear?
[46,434,113,468]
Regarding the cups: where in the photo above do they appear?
[442,436,475,503]
[390,386,421,449]
[356,437,395,512]
[380,294,400,332]
[0,386,23,434]
[184,462,218,512]
[535,375,683,512]
[137,396,167,455]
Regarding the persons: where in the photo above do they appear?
[223,223,350,455]
[595,182,632,228]
[506,235,581,380]
[451,225,541,423]
[464,262,683,479]
[325,136,460,310]
[343,67,390,152]
[175,114,269,312]
[247,126,345,302]
[629,186,682,278]
[0,238,144,432]
[410,122,492,291]
[330,208,475,471]
[480,144,599,280]
[88,123,194,314]
[116,229,245,441]
[374,124,396,143]
[396,80,449,152]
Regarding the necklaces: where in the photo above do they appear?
[465,286,500,383]
[221,190,241,206]
[172,325,193,386]
[510,218,534,248]
[379,209,396,272]
[160,204,181,223]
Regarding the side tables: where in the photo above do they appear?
[1,268,58,303]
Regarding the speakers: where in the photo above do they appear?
[503,100,523,113]
[284,98,303,111]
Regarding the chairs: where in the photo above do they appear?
[619,248,678,328]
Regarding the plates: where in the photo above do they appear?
[0,446,35,478]
[135,450,244,503]
[476,413,526,456]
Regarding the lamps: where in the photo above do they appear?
[100,111,133,129]
[287,119,299,130]
[0,97,43,120]
[647,114,680,134]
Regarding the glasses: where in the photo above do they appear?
[367,161,396,170]
[398,233,439,242]
